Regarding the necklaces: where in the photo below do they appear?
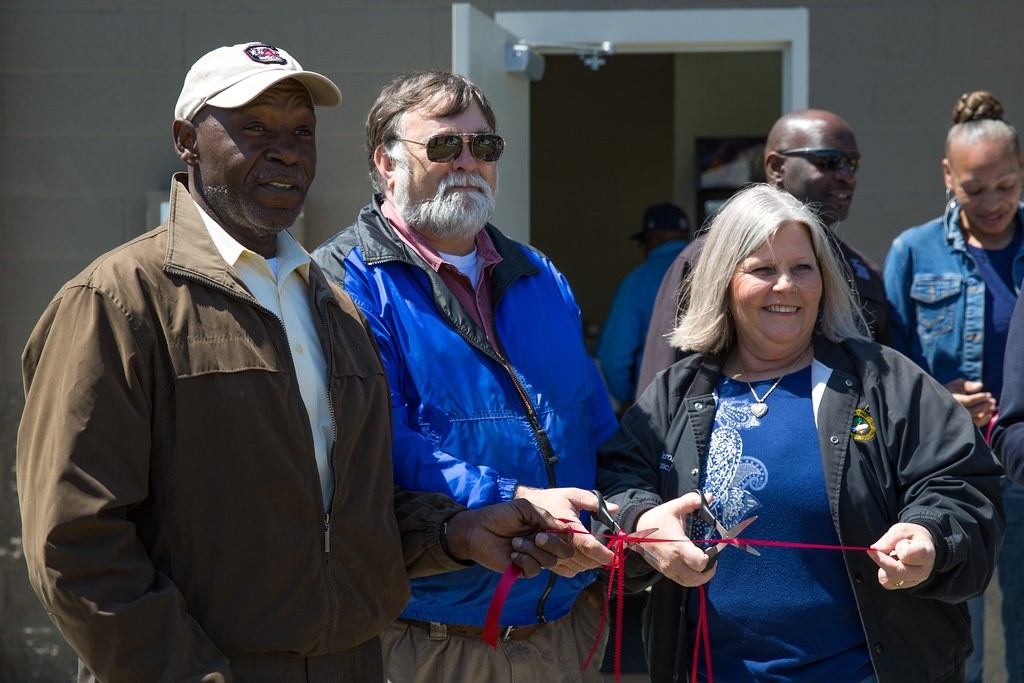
[736,340,813,419]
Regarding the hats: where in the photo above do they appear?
[174,41,342,123]
[629,203,689,240]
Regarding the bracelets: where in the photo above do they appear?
[440,513,478,566]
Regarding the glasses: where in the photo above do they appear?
[391,132,506,163]
[779,148,861,171]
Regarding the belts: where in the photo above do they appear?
[399,619,546,644]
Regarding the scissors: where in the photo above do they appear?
[589,490,662,565]
[691,489,761,573]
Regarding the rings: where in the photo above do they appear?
[895,580,905,587]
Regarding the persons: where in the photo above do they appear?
[309,70,619,683]
[596,90,1024,683]
[17,41,575,683]
[590,182,1007,683]
